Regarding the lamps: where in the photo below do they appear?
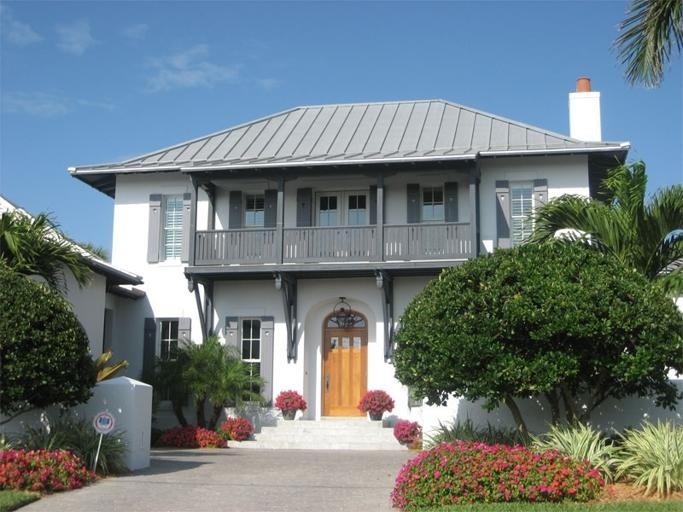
[334,296,350,328]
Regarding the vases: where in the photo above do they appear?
[369,409,383,420]
[282,409,296,420]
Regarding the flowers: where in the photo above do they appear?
[157,417,255,448]
[357,390,396,414]
[392,419,425,444]
[274,390,308,413]
